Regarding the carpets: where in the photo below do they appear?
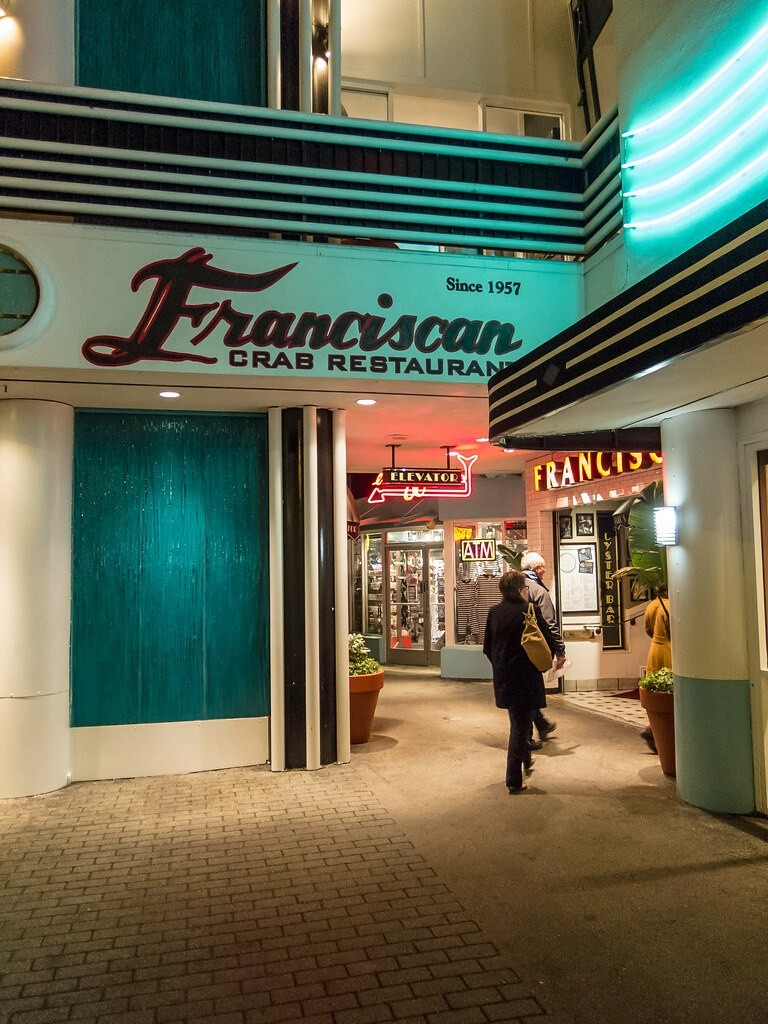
[604,687,640,700]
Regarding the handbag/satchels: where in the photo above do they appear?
[520,603,554,673]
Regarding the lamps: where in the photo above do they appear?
[653,506,678,547]
[426,521,435,529]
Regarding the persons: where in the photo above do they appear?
[483,571,562,794]
[642,584,673,675]
[520,552,568,750]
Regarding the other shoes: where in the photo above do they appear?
[529,738,544,750]
[641,726,657,755]
[539,720,556,740]
[508,782,528,793]
[524,756,536,770]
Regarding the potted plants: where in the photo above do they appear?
[349,632,385,744]
[637,667,676,780]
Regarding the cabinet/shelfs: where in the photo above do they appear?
[354,539,445,641]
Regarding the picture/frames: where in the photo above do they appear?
[576,512,594,536]
[626,559,637,576]
[630,579,649,601]
[559,515,573,539]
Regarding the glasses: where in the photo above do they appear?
[522,585,530,591]
